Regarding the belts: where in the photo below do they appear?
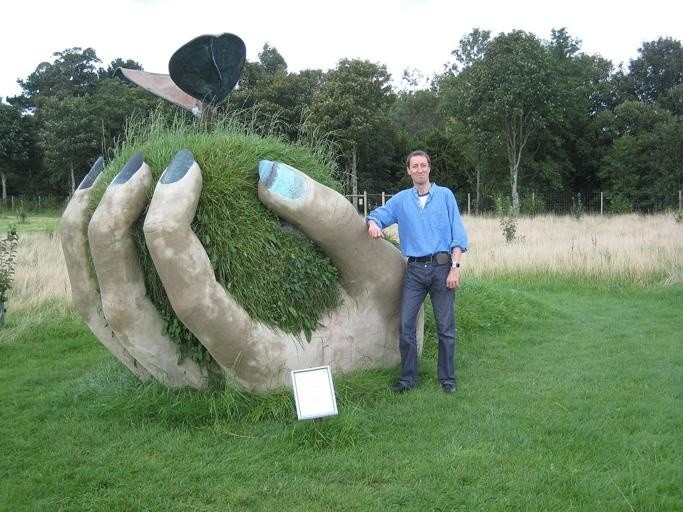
[408,256,433,264]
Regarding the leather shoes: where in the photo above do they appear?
[443,382,457,394]
[390,383,416,393]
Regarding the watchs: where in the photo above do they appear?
[450,263,461,270]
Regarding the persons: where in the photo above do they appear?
[362,149,472,397]
[57,145,426,397]
